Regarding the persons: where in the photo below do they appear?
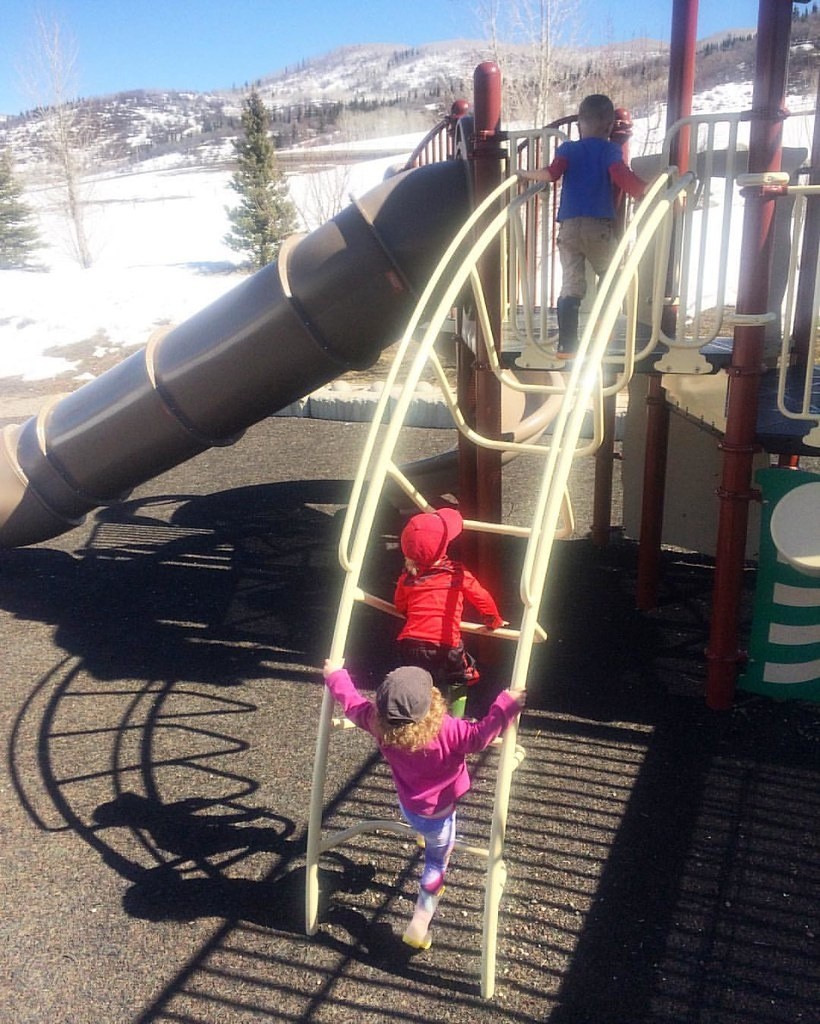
[514,94,650,359]
[394,508,510,724]
[323,659,527,948]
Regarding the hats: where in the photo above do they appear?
[375,665,434,726]
[401,508,464,564]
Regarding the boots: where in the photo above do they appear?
[402,884,447,950]
[556,294,581,359]
[416,832,425,849]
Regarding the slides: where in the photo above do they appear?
[0,158,472,549]
